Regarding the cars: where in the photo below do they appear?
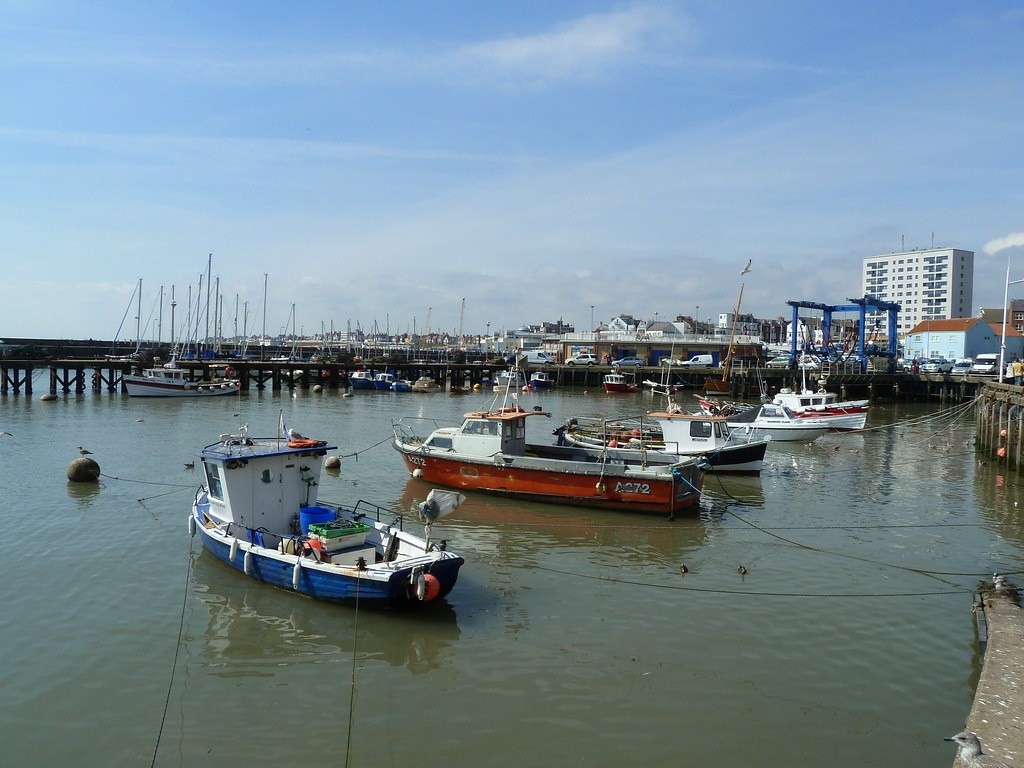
[921,359,952,373]
[798,355,822,370]
[951,362,973,374]
[611,356,646,368]
[896,359,912,372]
[766,357,789,369]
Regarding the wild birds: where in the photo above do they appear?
[287,429,305,440]
[77,446,94,458]
[0,431,13,437]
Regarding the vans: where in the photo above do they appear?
[521,350,554,364]
[971,353,1005,374]
[682,355,713,367]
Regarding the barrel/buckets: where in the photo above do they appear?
[299,507,336,535]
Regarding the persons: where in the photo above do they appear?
[1006,358,1024,386]
[607,353,612,366]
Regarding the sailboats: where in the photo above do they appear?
[642,329,684,393]
[104,252,476,375]
[703,283,745,396]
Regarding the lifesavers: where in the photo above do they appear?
[708,404,716,414]
[288,439,314,448]
[727,408,737,415]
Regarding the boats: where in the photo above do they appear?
[373,374,398,390]
[391,350,708,516]
[691,399,831,443]
[449,386,469,393]
[192,409,463,610]
[411,377,442,393]
[348,371,375,391]
[122,354,241,398]
[528,369,554,389]
[602,364,638,394]
[551,328,773,476]
[392,382,412,392]
[494,369,526,387]
[698,351,870,433]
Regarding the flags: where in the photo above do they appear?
[280,415,291,439]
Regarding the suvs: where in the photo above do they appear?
[565,354,599,366]
